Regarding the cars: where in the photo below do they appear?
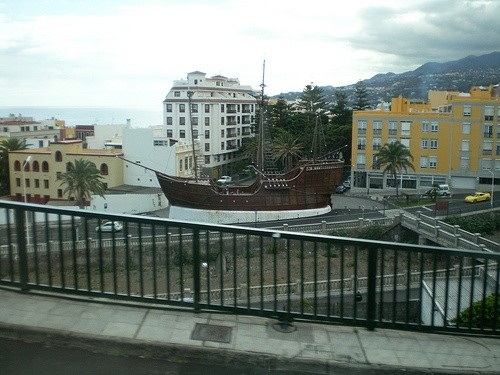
[216,175,232,184]
[336,185,345,193]
[344,181,352,187]
[95,221,124,232]
[464,191,491,202]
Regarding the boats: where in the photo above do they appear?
[155,159,344,223]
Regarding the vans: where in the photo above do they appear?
[431,184,451,196]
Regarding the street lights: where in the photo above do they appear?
[21,155,32,244]
